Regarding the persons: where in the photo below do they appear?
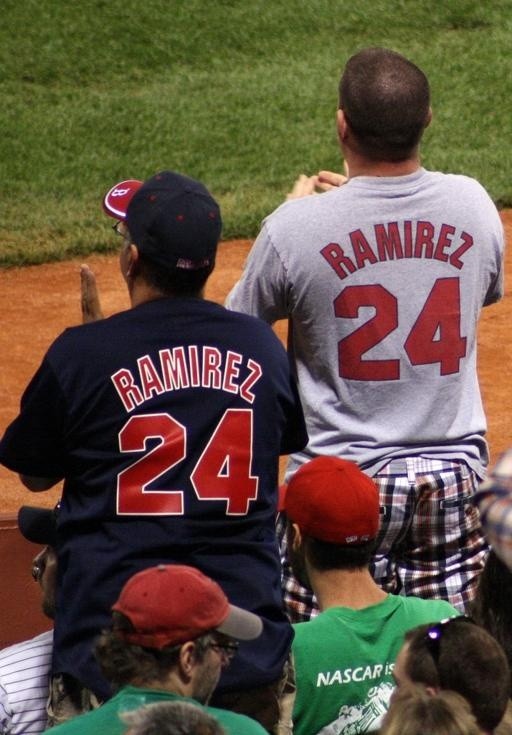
[389,617,512,734]
[227,46,503,622]
[37,563,277,735]
[0,499,70,733]
[0,168,309,734]
[272,455,461,735]
[118,700,220,735]
[376,686,483,735]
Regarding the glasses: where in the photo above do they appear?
[112,220,130,242]
[163,638,239,659]
[424,613,476,688]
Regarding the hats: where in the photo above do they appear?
[277,456,380,543]
[18,500,61,557]
[112,565,263,646]
[102,170,220,268]
